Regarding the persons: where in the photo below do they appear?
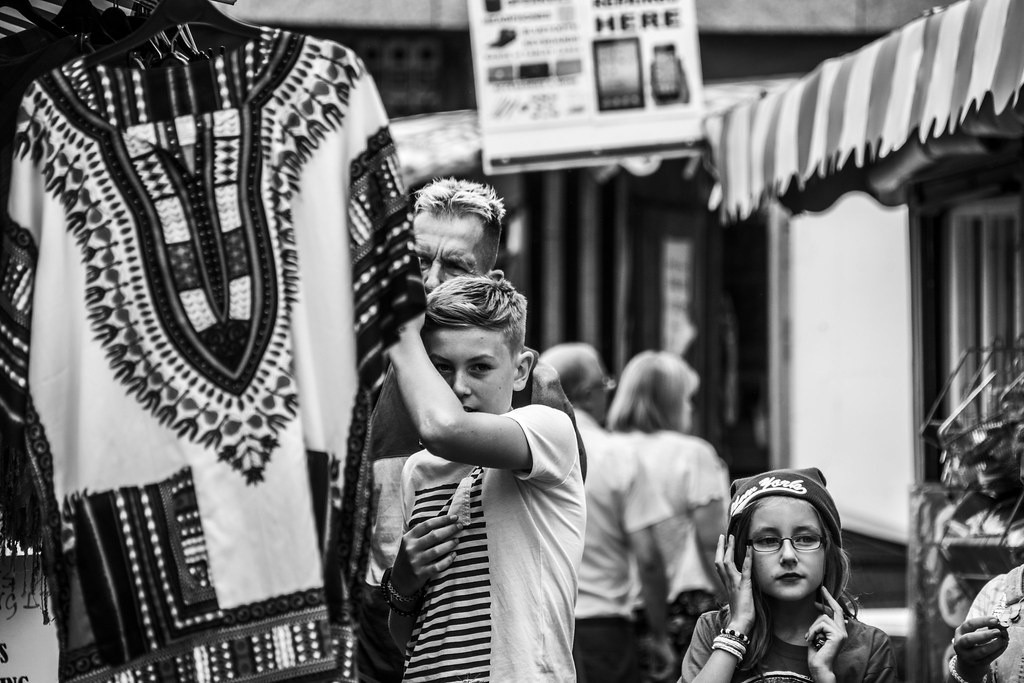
[539,343,728,683]
[677,467,897,683]
[354,180,588,683]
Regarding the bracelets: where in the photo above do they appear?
[381,565,422,618]
[948,655,968,683]
[711,628,750,662]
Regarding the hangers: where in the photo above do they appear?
[937,342,1024,455]
[0,1,276,67]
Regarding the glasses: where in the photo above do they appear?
[601,377,615,390]
[743,532,825,553]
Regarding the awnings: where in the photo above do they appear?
[714,1,1023,226]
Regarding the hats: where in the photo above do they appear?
[725,468,843,549]
